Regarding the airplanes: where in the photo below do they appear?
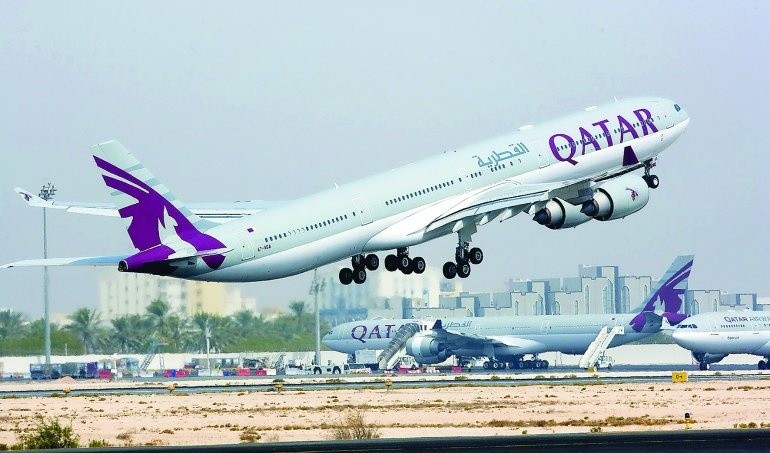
[670,309,769,372]
[1,95,691,287]
[322,254,694,370]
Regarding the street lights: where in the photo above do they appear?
[35,181,59,366]
